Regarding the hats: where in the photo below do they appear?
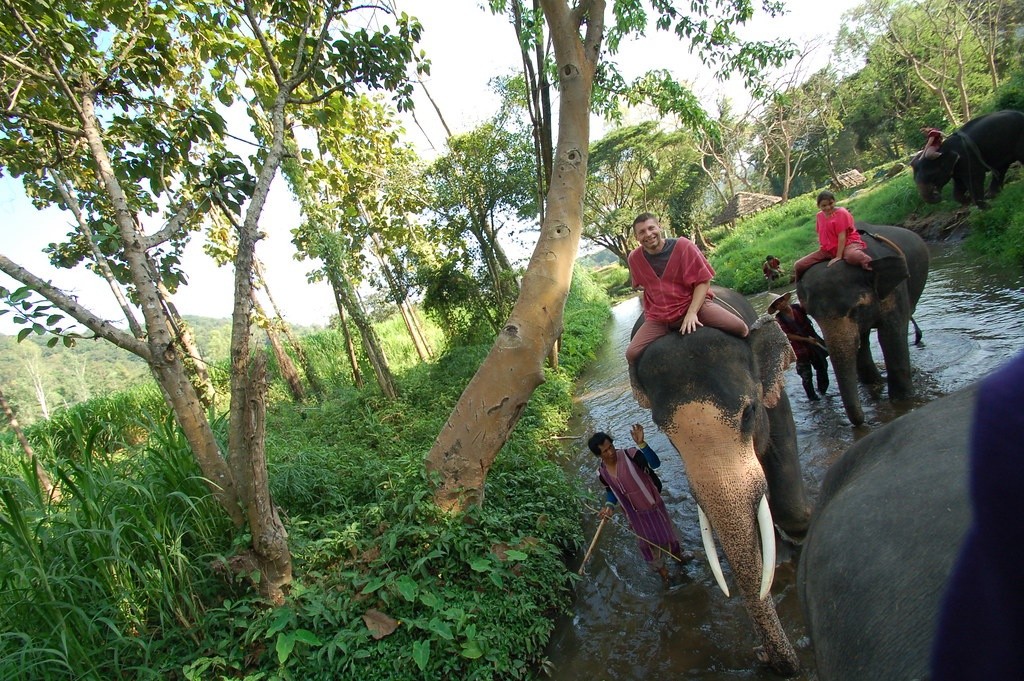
[767,289,797,315]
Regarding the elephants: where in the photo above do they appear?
[911,109,1024,206]
[796,346,1023,681]
[794,220,931,429]
[629,287,815,677]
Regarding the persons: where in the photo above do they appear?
[794,191,873,286]
[763,255,780,290]
[929,352,1024,681]
[625,213,749,364]
[587,423,693,590]
[765,292,830,400]
[919,125,944,161]
